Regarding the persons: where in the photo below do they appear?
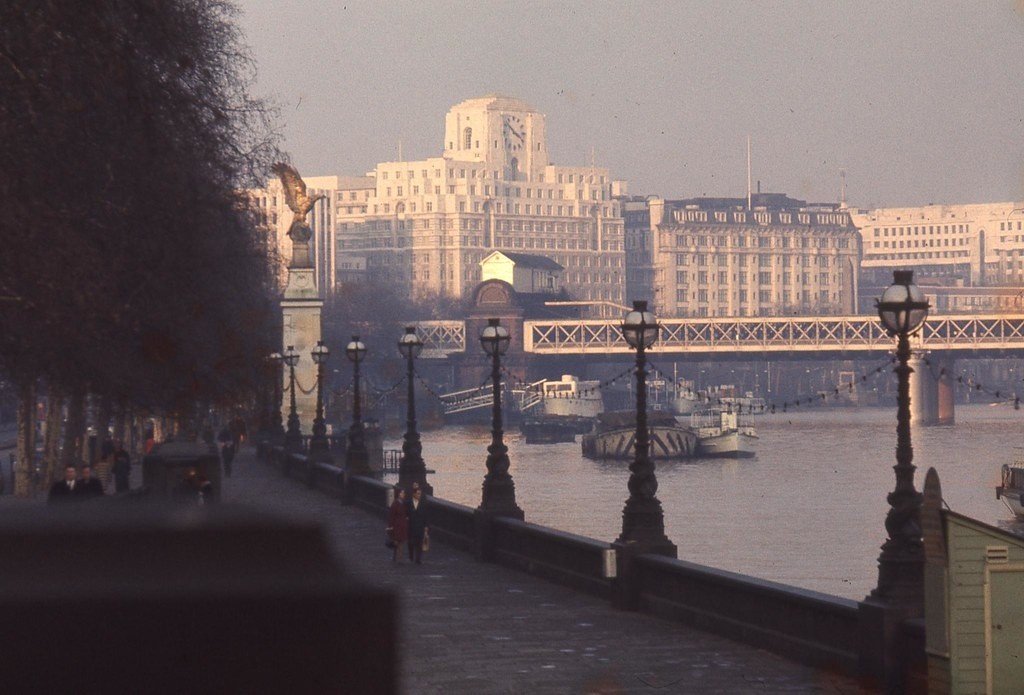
[47,465,80,514]
[222,436,235,478]
[77,465,103,500]
[406,488,428,566]
[111,442,130,491]
[410,480,426,500]
[197,474,214,508]
[188,478,204,509]
[389,486,409,564]
[99,427,241,466]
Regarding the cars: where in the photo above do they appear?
[1,412,150,484]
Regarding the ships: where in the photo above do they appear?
[542,372,606,433]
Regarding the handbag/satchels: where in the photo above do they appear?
[385,529,400,549]
[422,532,430,551]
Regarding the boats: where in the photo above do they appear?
[521,410,576,445]
[691,407,758,457]
[997,455,1024,520]
[581,369,696,460]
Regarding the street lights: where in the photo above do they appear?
[268,351,284,440]
[398,327,433,488]
[472,319,526,516]
[284,347,303,451]
[613,301,669,552]
[345,335,372,473]
[867,270,930,612]
[310,340,331,458]
